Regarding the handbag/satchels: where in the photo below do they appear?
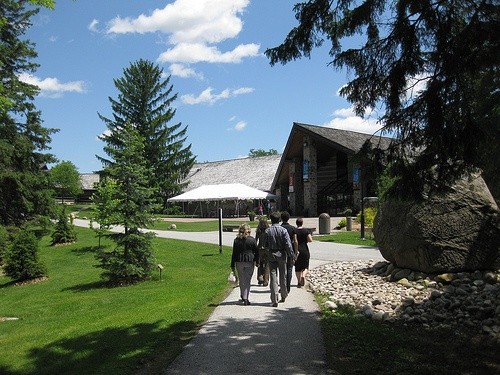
[228,271,238,287]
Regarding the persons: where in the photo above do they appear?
[230,211,313,307]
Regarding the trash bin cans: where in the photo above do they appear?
[319,213,330,234]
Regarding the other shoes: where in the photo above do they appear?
[281,297,285,302]
[272,303,278,307]
[300,277,304,286]
[239,299,250,305]
[297,283,301,288]
[258,281,264,284]
[263,283,268,286]
